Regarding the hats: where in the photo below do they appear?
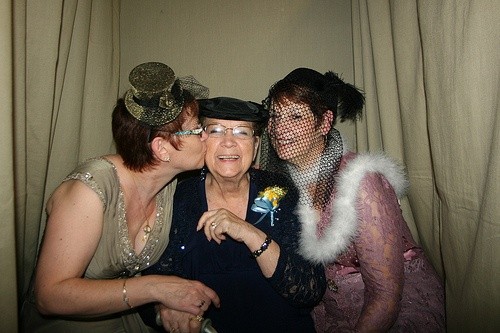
[283,68,338,123]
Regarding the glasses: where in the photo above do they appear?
[171,123,204,139]
[204,123,256,136]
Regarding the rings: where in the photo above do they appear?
[199,300,205,308]
[211,222,217,228]
[196,316,203,321]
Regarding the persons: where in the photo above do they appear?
[139,96,327,333]
[258,67,446,333]
[18,62,219,333]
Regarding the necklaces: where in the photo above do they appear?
[131,176,152,235]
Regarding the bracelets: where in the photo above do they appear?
[122,278,134,308]
[253,235,272,258]
